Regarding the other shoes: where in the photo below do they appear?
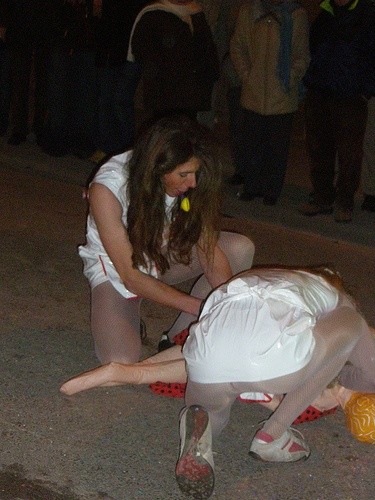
[157,330,177,354]
[262,191,278,206]
[7,134,26,145]
[139,318,148,340]
[299,202,334,216]
[231,174,246,185]
[338,205,354,222]
[237,189,263,201]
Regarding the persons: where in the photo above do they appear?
[59,328,356,426]
[0,1,374,225]
[174,261,375,499]
[77,117,255,365]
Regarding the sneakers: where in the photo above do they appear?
[248,421,311,463]
[175,404,215,500]
